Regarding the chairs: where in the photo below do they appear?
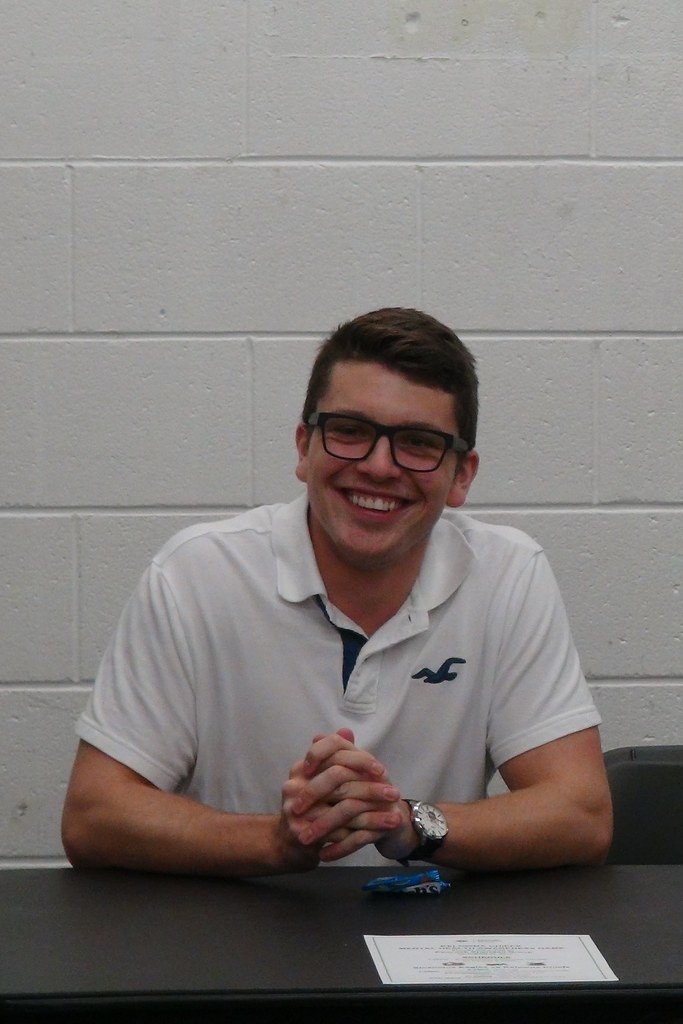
[598,742,683,865]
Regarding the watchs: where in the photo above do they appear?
[397,798,449,869]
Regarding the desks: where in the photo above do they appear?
[0,865,683,1024]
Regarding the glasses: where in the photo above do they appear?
[307,411,467,472]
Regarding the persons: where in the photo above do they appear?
[61,307,614,880]
